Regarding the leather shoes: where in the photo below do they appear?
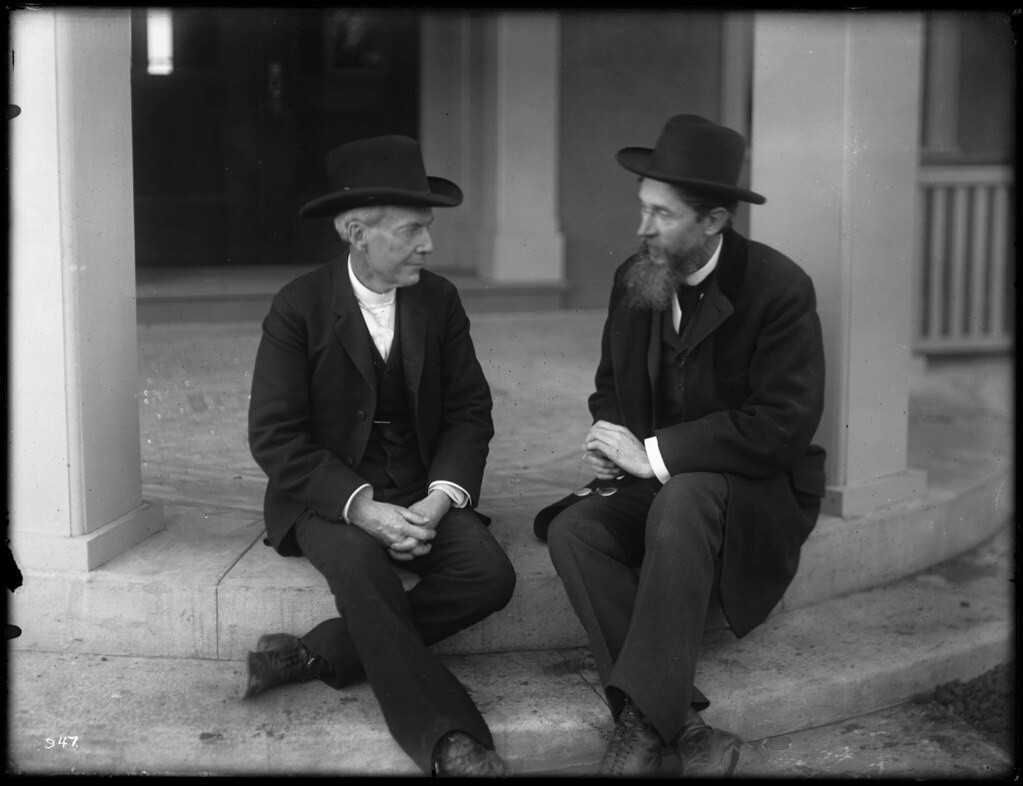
[680,727,741,776]
[434,733,505,778]
[597,703,662,775]
[238,634,317,699]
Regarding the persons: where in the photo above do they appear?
[242,128,519,777]
[535,114,830,777]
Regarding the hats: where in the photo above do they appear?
[298,135,463,217]
[615,114,766,205]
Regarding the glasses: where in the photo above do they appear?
[571,452,620,497]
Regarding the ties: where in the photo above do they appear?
[676,269,715,338]
